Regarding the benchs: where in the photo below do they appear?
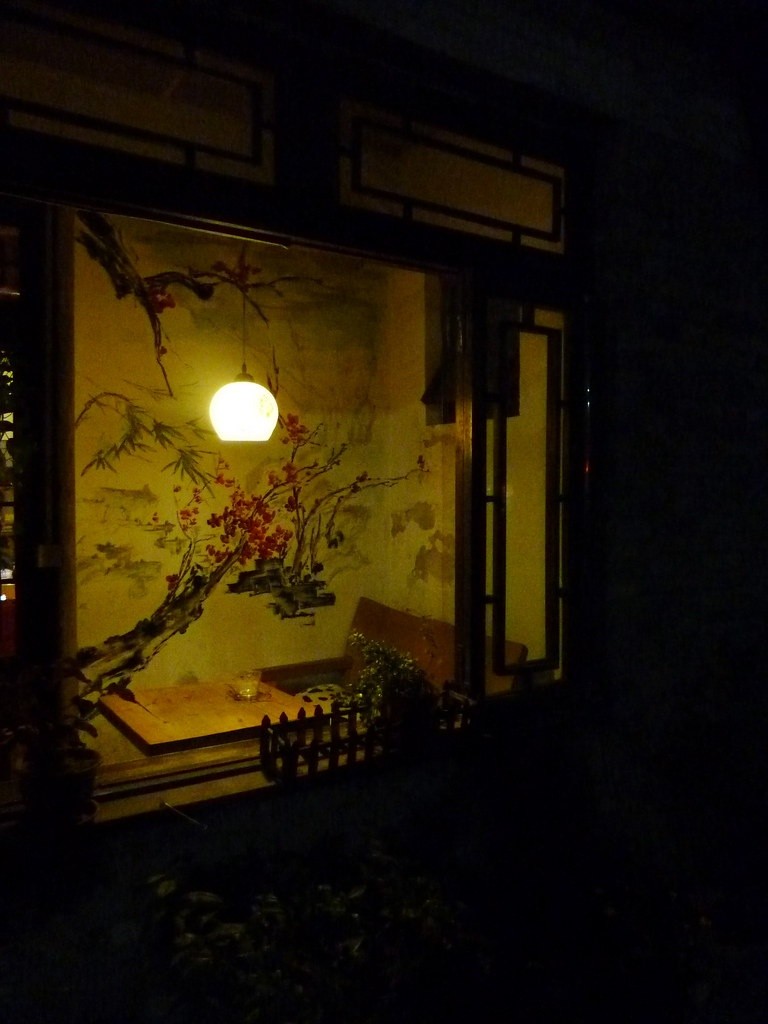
[260,597,529,696]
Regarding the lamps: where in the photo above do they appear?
[209,254,279,442]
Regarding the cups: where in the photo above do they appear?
[226,670,261,698]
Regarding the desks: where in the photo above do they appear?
[98,680,332,757]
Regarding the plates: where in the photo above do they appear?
[229,693,272,702]
